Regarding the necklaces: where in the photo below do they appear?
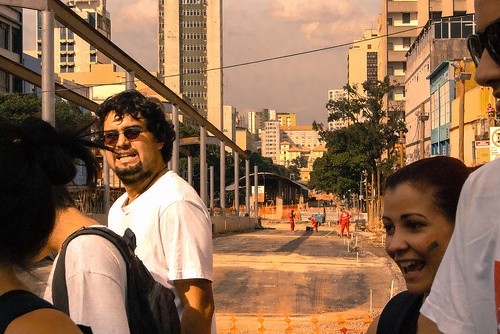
[122,165,169,206]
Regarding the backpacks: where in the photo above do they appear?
[51,227,183,334]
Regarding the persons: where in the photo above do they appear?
[95,89,216,334]
[289,209,295,231]
[0,138,84,334]
[416,0,499,333]
[365,155,483,333]
[338,207,351,238]
[13,117,132,334]
[308,217,319,232]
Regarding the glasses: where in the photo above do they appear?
[104,128,148,144]
[468,17,500,66]
